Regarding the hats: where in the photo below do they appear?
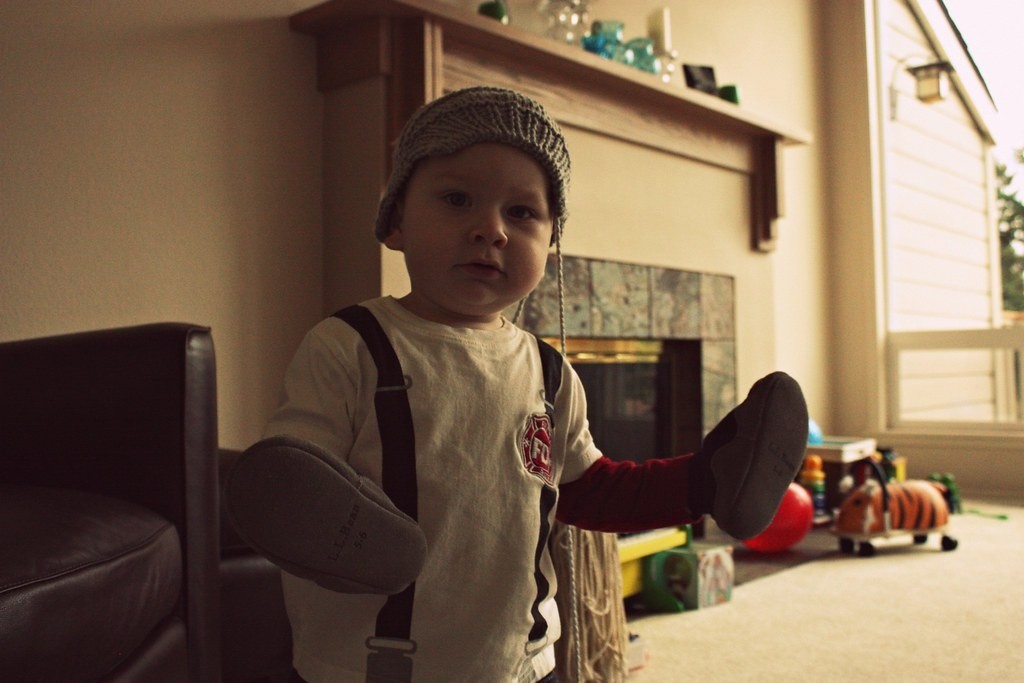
[374,87,571,246]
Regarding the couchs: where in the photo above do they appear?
[0,321,302,683]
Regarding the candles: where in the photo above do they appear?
[647,8,672,52]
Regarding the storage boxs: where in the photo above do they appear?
[804,435,878,505]
[666,543,735,610]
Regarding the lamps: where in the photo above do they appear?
[886,51,958,119]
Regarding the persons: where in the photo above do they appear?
[225,87,807,683]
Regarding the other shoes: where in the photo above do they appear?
[226,433,429,595]
[697,372,806,541]
